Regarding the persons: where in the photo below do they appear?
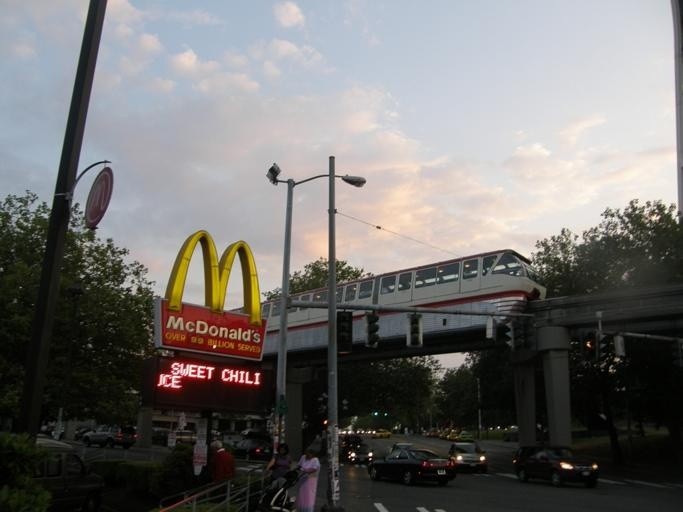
[206,441,236,502]
[285,446,321,510]
[262,441,293,491]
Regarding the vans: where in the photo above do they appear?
[17,434,109,510]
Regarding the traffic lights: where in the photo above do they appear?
[404,312,423,349]
[596,332,609,362]
[365,309,380,349]
[494,321,513,352]
[335,310,354,356]
[582,337,594,361]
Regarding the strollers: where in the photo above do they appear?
[253,467,306,512]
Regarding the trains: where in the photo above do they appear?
[254,246,550,336]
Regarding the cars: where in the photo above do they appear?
[367,449,456,485]
[448,441,488,472]
[172,430,199,446]
[383,441,417,460]
[342,434,363,446]
[439,430,474,443]
[341,445,375,465]
[152,426,170,442]
[72,425,136,449]
[218,429,244,446]
[423,430,440,438]
[371,428,392,439]
[512,444,597,486]
[240,427,259,436]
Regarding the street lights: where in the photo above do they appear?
[263,161,367,460]
